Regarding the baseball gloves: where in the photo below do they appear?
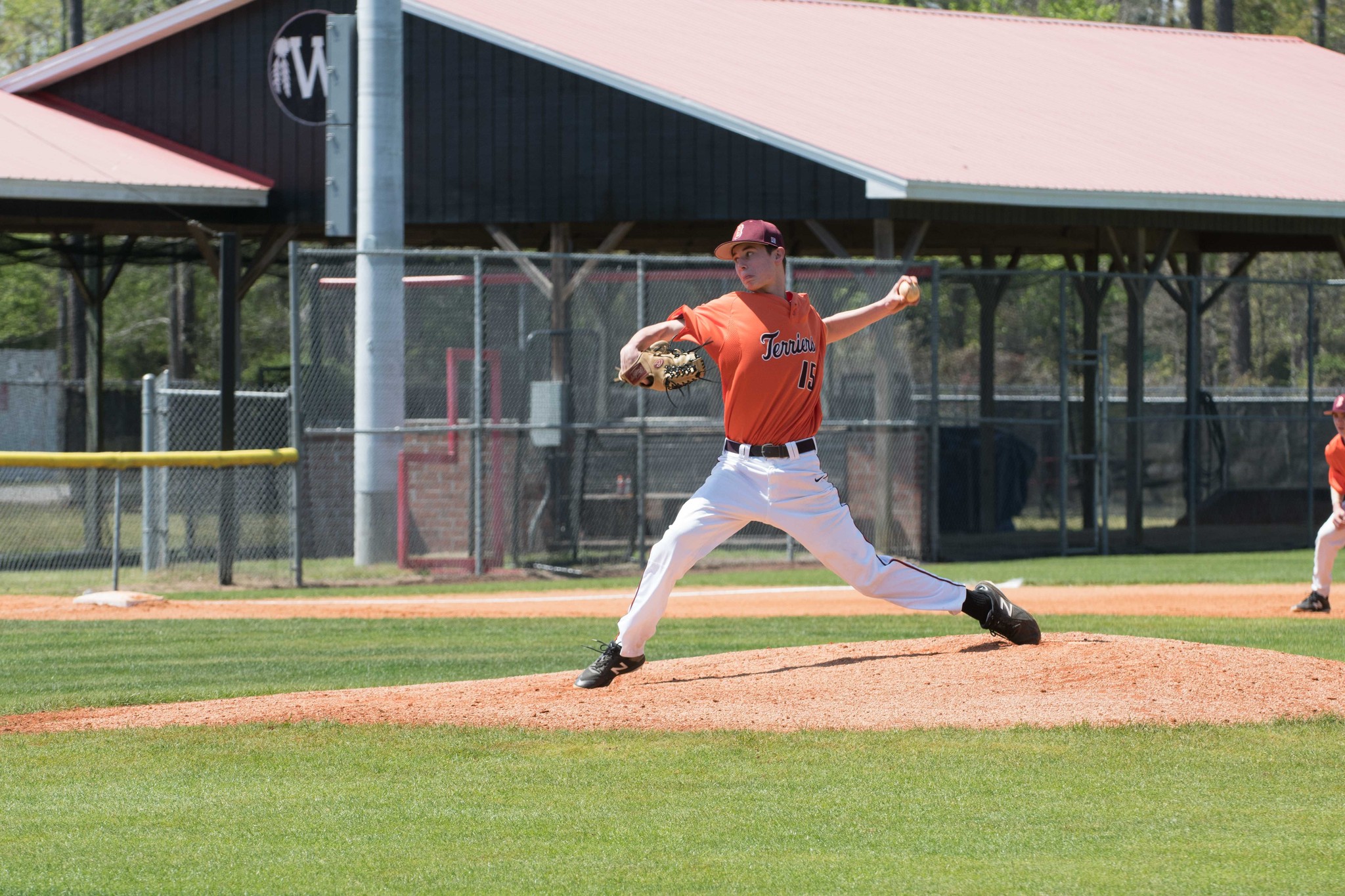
[621,340,706,392]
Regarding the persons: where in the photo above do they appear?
[1290,393,1345,610]
[572,220,1040,690]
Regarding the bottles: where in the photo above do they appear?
[617,475,624,494]
[624,476,632,494]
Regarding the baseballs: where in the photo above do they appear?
[899,280,920,302]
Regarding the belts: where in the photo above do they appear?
[724,439,815,459]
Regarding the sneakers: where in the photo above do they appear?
[1291,590,1331,612]
[974,581,1041,645]
[573,639,645,689]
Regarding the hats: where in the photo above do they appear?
[1324,393,1345,415]
[714,220,784,260]
